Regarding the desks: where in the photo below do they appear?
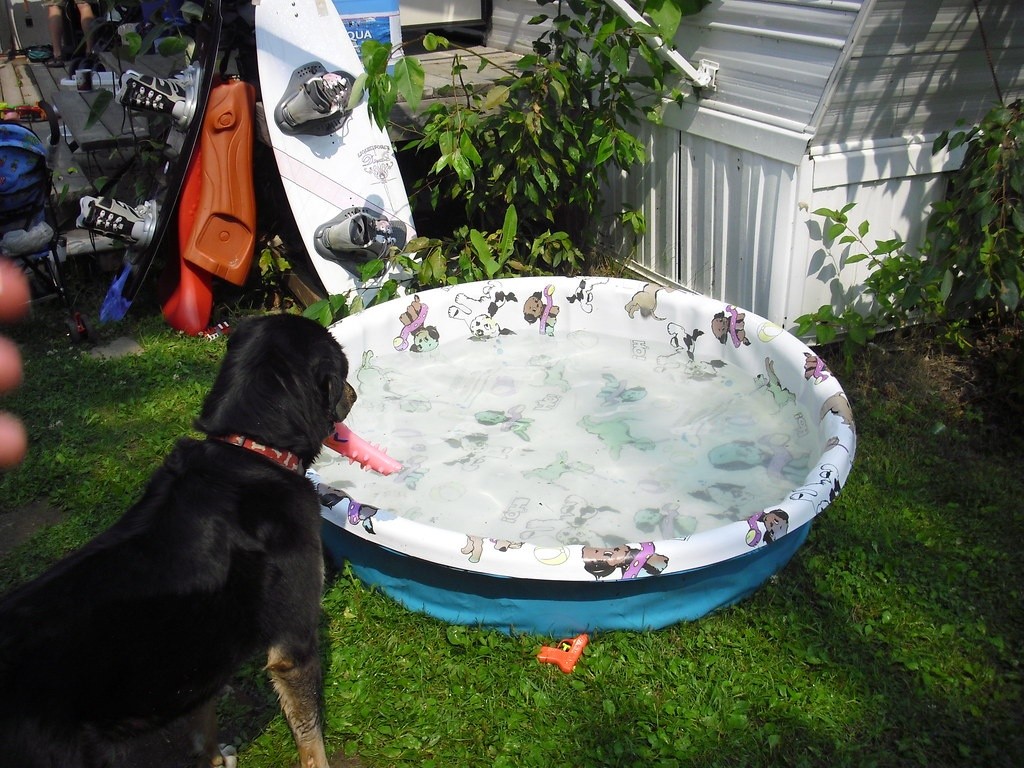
[69,51,237,198]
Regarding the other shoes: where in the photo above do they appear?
[284,72,355,127]
[322,211,396,261]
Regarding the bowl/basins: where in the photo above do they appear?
[305,274,858,583]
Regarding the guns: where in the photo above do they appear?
[537,634,589,674]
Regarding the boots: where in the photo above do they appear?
[113,67,185,121]
[74,195,143,254]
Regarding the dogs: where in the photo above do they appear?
[0,313,360,768]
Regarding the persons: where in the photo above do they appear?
[0,258,31,466]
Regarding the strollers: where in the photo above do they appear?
[0,101,89,345]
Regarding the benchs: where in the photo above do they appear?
[51,89,151,154]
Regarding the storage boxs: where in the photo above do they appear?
[330,0,405,79]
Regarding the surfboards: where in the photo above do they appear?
[253,0,424,315]
[99,0,221,322]
